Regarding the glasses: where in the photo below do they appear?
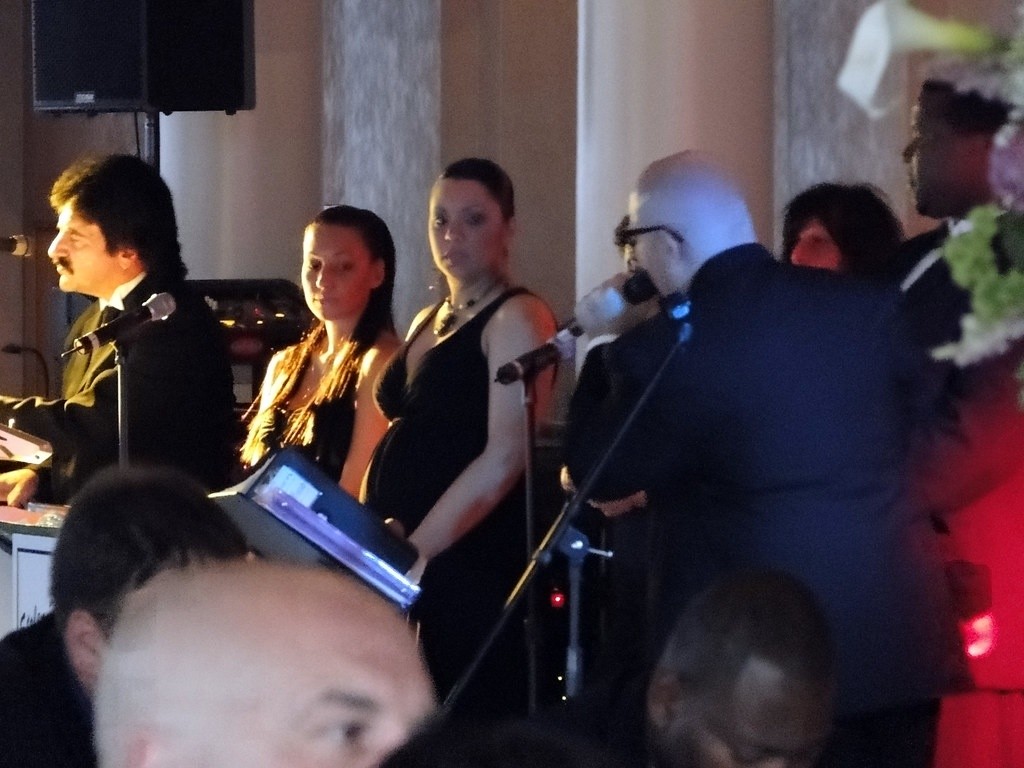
[613,214,685,245]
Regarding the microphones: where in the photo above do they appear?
[62,293,177,359]
[494,336,574,386]
[556,273,657,344]
[0,235,33,257]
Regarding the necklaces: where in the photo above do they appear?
[434,278,508,336]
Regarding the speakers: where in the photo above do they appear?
[31,0,256,116]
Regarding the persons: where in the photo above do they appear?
[0,76,1024,768]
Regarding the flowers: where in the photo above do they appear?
[835,0,1024,368]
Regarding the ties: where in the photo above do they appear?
[98,305,123,327]
